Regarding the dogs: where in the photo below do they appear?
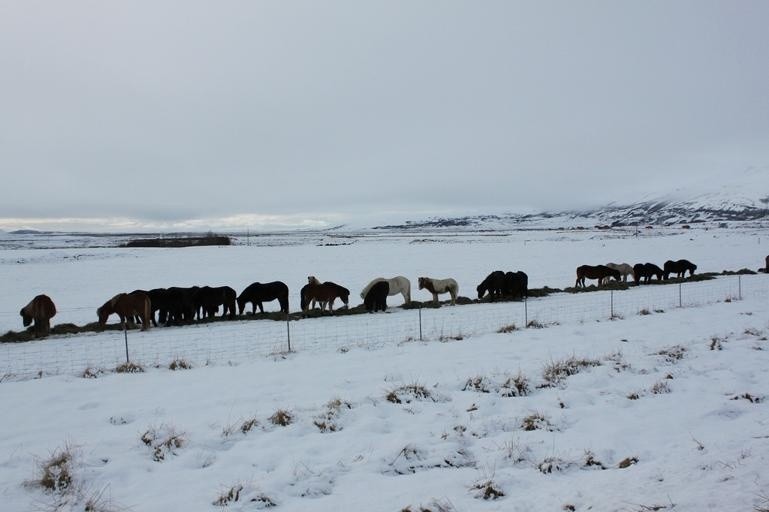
[417,276,459,307]
[476,270,528,302]
[235,281,289,314]
[575,259,697,288]
[95,285,237,332]
[359,275,411,313]
[19,294,56,338]
[300,276,350,318]
